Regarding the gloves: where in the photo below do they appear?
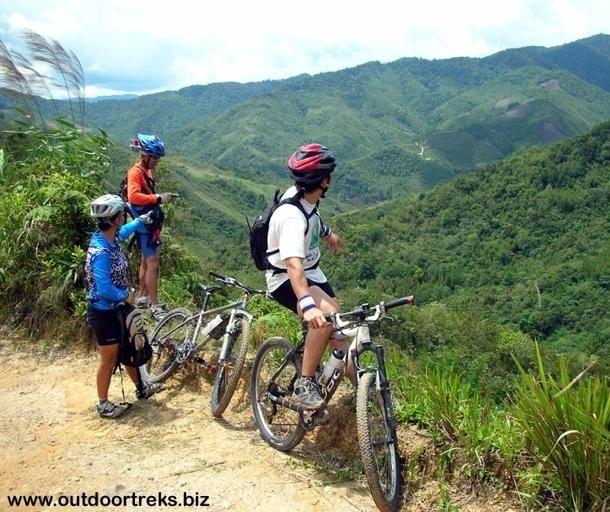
[160,192,174,204]
[139,210,154,225]
[126,292,135,304]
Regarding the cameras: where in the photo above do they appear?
[167,193,179,200]
[134,296,147,309]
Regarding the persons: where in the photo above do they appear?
[265,141,401,409]
[84,193,167,420]
[127,133,172,324]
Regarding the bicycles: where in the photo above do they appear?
[137,268,268,420]
[249,293,420,512]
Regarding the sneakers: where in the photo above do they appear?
[135,381,161,400]
[96,400,127,418]
[136,296,167,322]
[291,377,326,411]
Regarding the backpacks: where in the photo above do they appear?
[246,188,320,277]
[112,300,153,375]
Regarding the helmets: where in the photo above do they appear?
[287,143,337,185]
[90,194,126,218]
[137,134,165,158]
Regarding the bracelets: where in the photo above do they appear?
[322,227,332,240]
[298,296,317,312]
